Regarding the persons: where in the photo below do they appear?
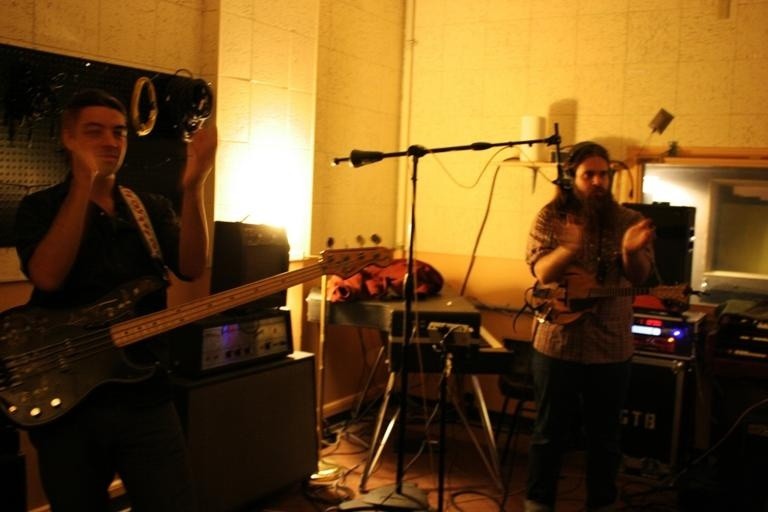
[13,88,218,511]
[524,141,655,512]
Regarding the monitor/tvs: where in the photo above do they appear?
[688,177,768,295]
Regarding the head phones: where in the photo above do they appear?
[561,142,595,190]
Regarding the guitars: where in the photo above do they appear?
[522,265,714,327]
[0,234,392,432]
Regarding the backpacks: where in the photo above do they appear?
[327,258,444,304]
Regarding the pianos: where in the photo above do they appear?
[304,284,506,492]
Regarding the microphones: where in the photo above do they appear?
[555,123,564,178]
